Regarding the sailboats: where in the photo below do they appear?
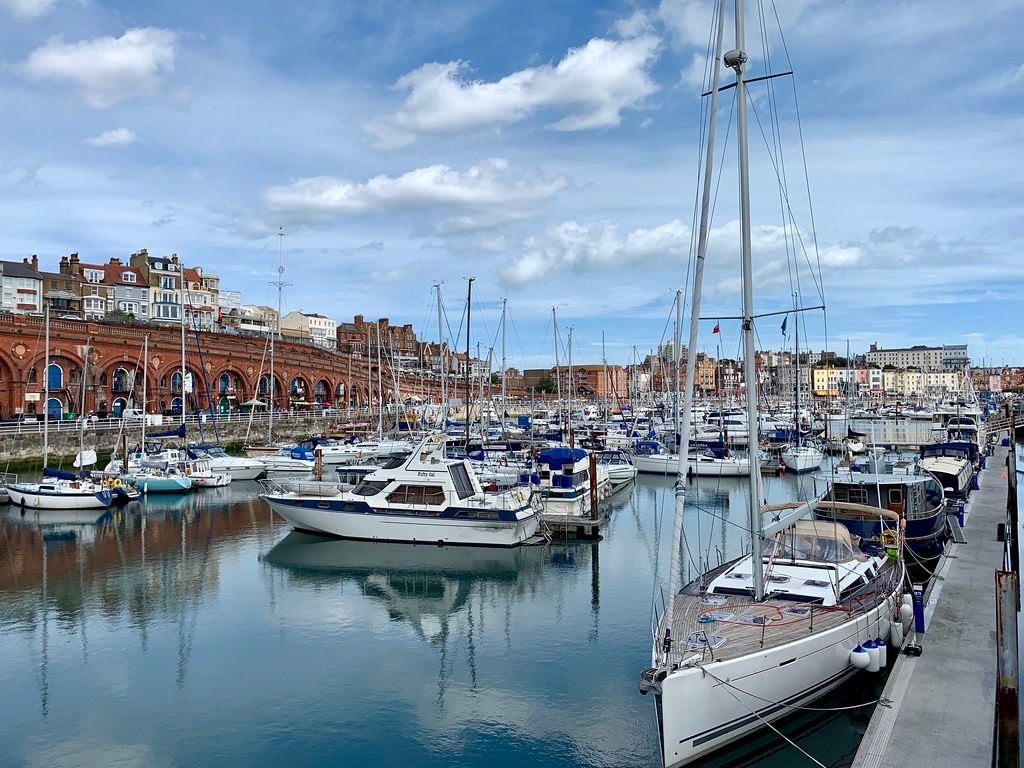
[0,1,981,566]
[638,1,915,768]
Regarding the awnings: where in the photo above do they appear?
[293,400,311,408]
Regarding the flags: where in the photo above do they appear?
[713,325,720,334]
[781,317,788,335]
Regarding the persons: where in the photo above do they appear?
[293,406,312,411]
[342,400,349,411]
[273,405,289,415]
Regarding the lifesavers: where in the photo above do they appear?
[880,529,897,545]
[186,468,190,476]
[114,479,122,487]
[609,442,615,447]
[517,490,525,502]
[434,438,439,443]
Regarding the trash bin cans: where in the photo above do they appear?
[63,413,77,422]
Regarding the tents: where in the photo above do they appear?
[240,398,268,414]
[310,401,322,409]
[322,402,331,410]
